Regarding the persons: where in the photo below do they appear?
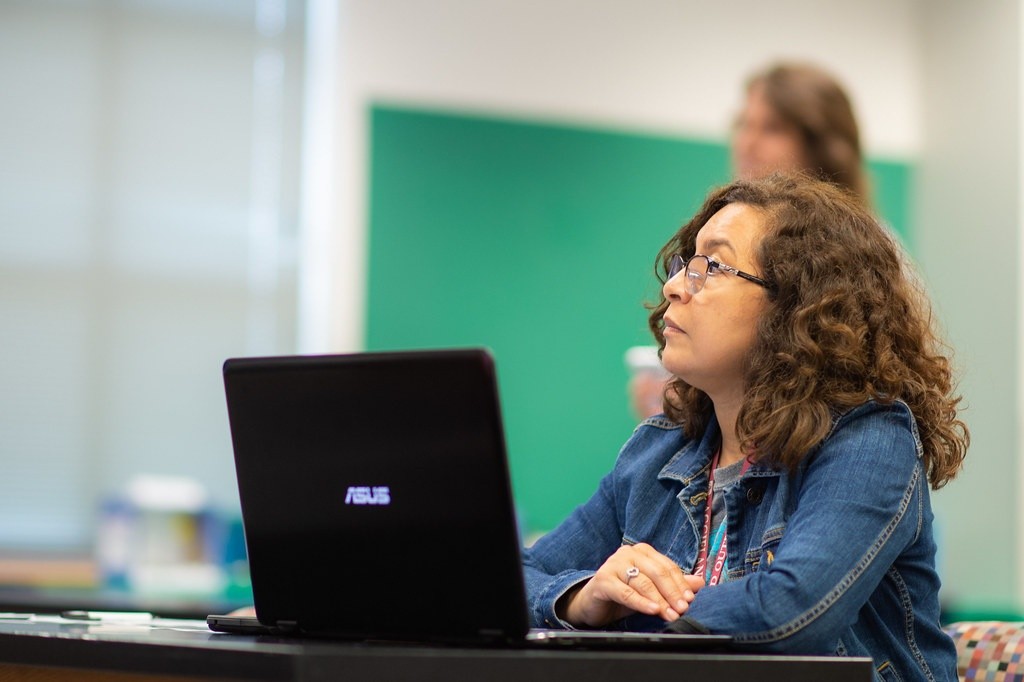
[518,169,973,681]
[728,56,870,213]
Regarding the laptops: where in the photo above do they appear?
[223,347,735,652]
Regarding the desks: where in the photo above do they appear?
[0,614,875,682]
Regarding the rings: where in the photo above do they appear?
[626,565,639,586]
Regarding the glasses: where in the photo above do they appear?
[667,255,774,295]
